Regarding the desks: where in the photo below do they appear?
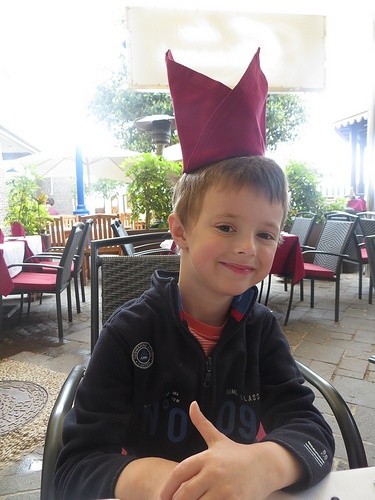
[295,467,375,500]
[2,235,48,275]
[260,233,307,326]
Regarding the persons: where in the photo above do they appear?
[44,198,60,215]
[53,154,336,500]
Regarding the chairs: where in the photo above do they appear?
[0,208,375,500]
